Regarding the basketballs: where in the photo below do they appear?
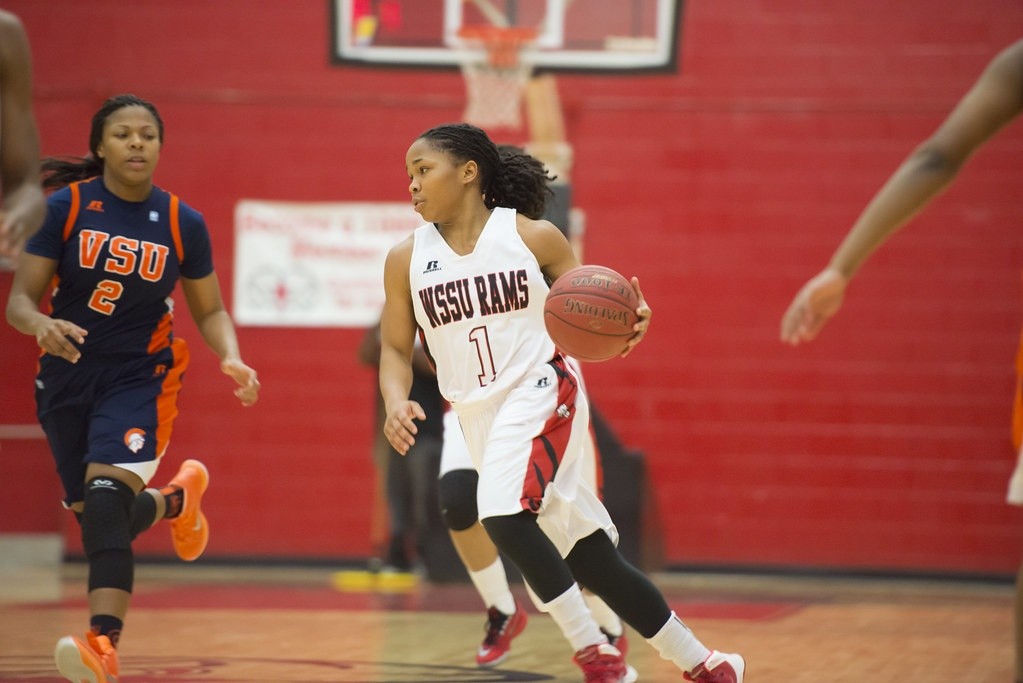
[543,264,639,363]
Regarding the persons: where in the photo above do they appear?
[361,122,744,683]
[782,42,1023,683]
[7,94,261,683]
[0,11,48,258]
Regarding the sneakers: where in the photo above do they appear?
[572,644,639,683]
[476,598,528,668]
[682,650,746,683]
[53,632,120,683]
[166,459,210,562]
[599,623,629,658]
